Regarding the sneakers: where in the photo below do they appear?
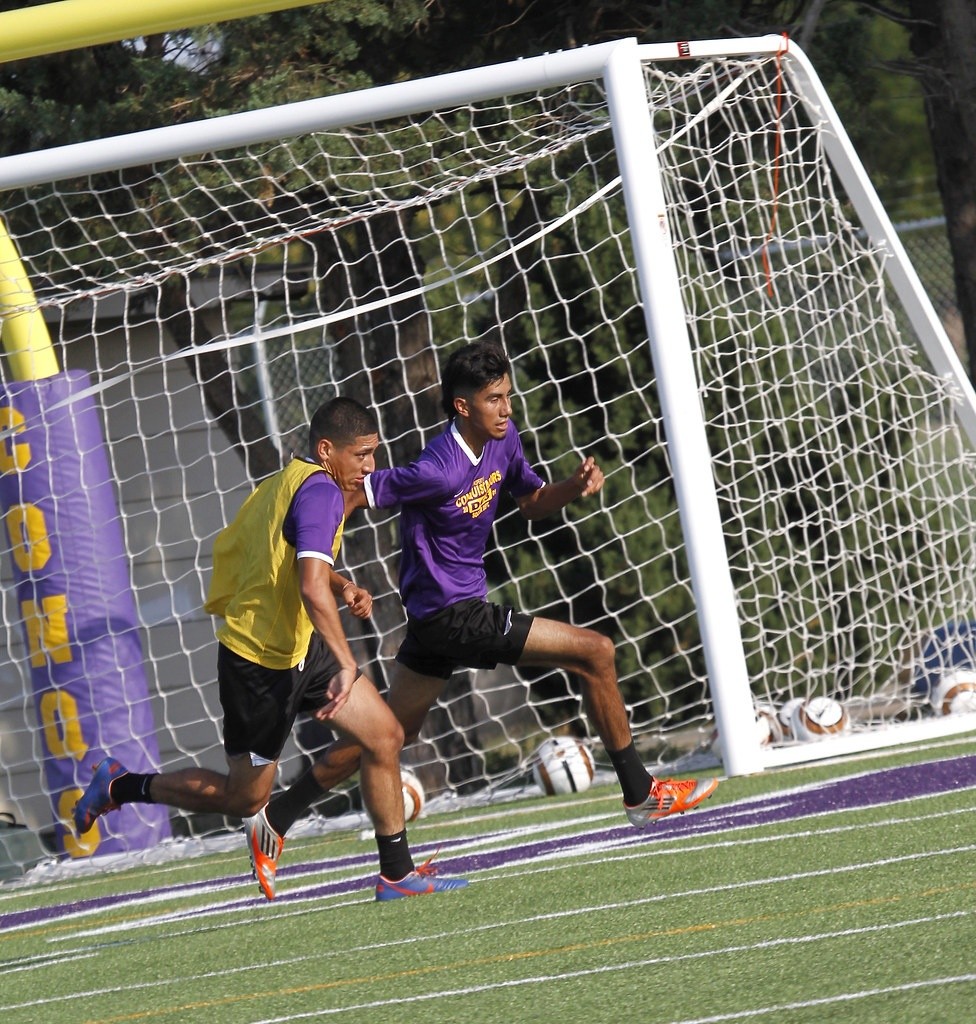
[242,803,285,904]
[70,756,129,833]
[624,775,719,831]
[375,845,469,903]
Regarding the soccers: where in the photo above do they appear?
[793,697,853,743]
[929,668,976,723]
[364,761,426,829]
[710,697,807,761]
[533,736,598,797]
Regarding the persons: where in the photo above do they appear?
[240,345,720,902]
[72,398,468,902]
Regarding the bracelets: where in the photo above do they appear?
[341,581,355,590]
